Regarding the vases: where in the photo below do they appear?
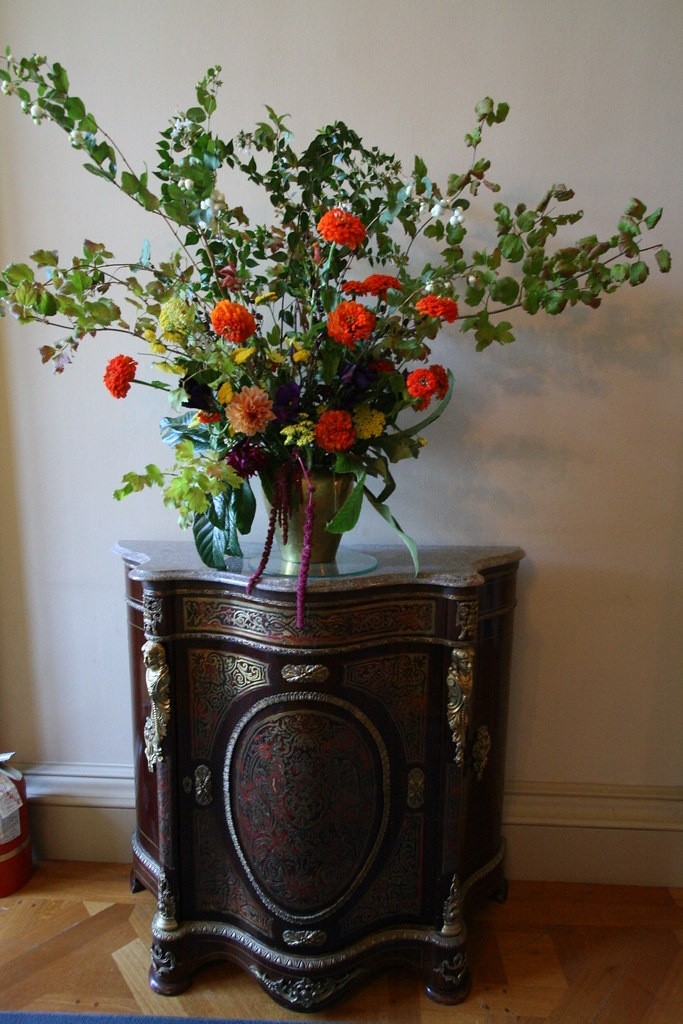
[267,472,352,563]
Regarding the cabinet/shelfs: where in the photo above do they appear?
[118,540,527,1013]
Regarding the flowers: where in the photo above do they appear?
[0,52,672,511]
